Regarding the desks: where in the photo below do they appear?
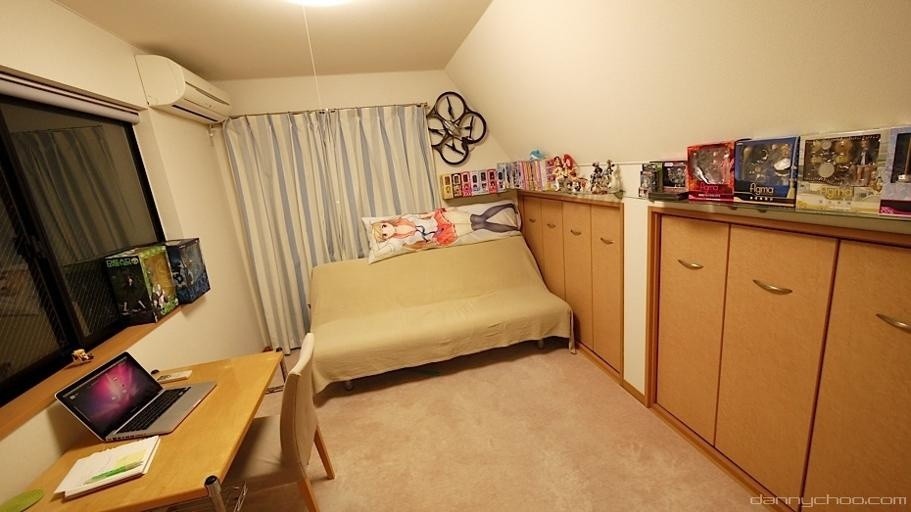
[0,350,298,512]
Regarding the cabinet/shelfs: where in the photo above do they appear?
[642,200,910,511]
[514,187,623,394]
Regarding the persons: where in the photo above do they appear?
[435,121,911,221]
[369,201,523,251]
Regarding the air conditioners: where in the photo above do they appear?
[134,51,233,127]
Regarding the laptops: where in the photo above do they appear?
[53,352,216,443]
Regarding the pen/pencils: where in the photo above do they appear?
[83,460,143,485]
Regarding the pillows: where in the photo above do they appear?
[360,198,521,265]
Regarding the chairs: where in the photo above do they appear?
[223,328,341,512]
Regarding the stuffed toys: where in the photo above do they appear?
[435,121,911,221]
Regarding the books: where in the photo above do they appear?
[52,433,163,502]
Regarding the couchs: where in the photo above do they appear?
[307,233,578,400]
